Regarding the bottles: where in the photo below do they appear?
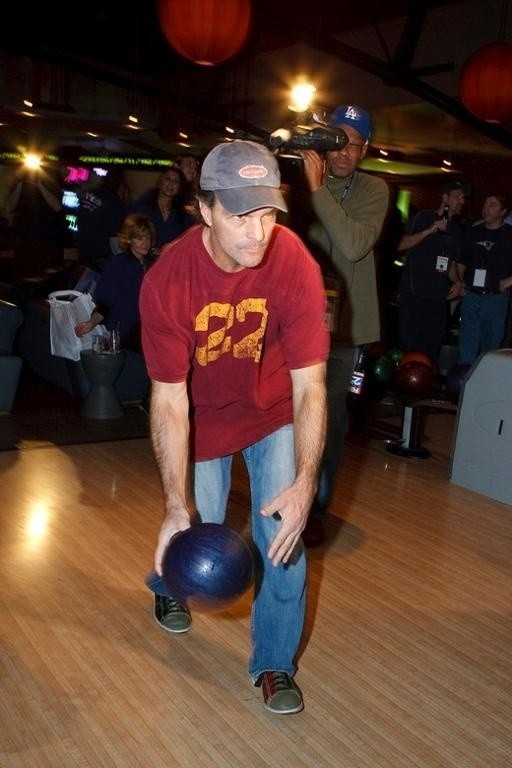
[437,206,448,239]
[344,352,366,404]
[92,329,121,356]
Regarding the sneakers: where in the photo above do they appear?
[151,587,195,637]
[255,668,307,717]
[302,514,325,548]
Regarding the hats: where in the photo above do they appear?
[195,136,292,221]
[328,102,371,140]
[441,181,469,196]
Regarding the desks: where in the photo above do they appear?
[83,349,129,421]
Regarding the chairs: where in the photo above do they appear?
[43,286,145,405]
[0,309,26,419]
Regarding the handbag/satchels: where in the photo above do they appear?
[41,288,114,361]
